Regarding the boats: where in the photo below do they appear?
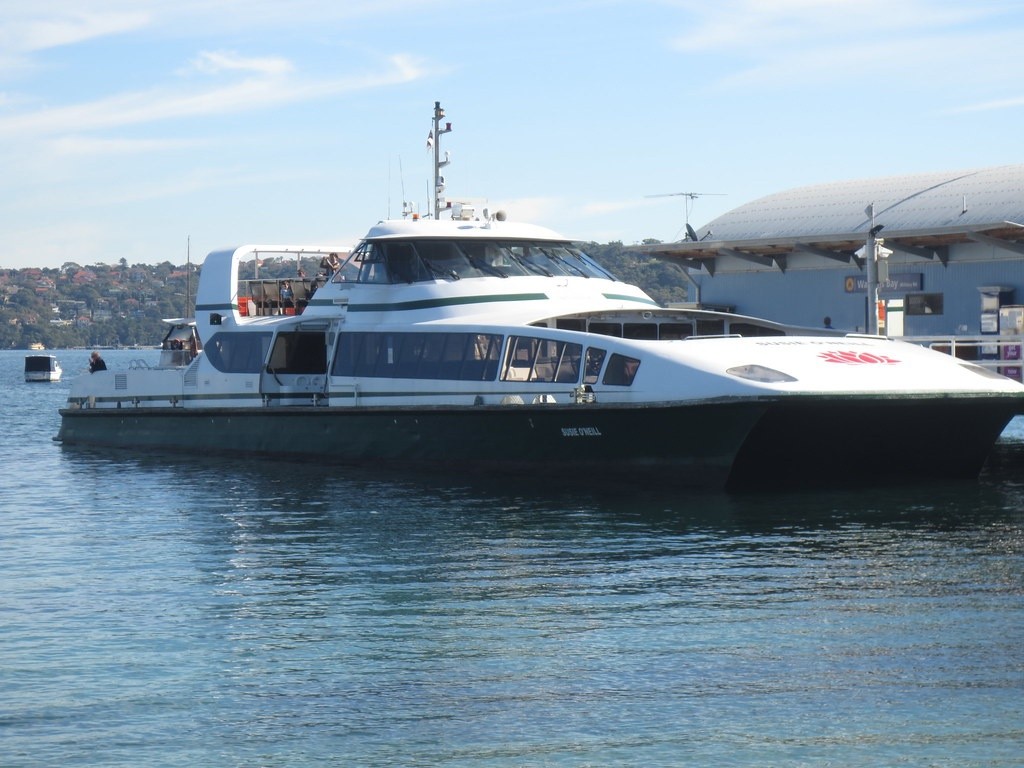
[25,355,61,382]
[26,342,45,350]
[50,97,1024,493]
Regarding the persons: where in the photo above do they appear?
[308,283,318,302]
[173,336,198,365]
[298,269,305,277]
[89,352,108,373]
[280,281,297,309]
[321,253,341,282]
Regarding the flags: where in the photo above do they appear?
[427,131,433,149]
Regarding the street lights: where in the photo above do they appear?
[853,224,894,335]
